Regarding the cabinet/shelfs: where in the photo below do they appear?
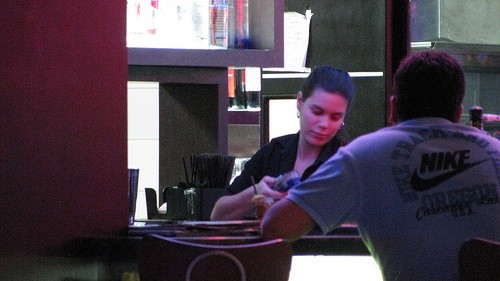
[385,0,500,134]
[127,2,284,192]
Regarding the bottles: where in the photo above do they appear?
[208,1,229,50]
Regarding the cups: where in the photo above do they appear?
[470,106,483,131]
[252,194,275,220]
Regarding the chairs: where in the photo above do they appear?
[136,230,292,280]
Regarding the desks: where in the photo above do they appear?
[0,227,384,280]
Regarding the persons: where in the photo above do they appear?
[260,50,500,281]
[210,65,353,221]
